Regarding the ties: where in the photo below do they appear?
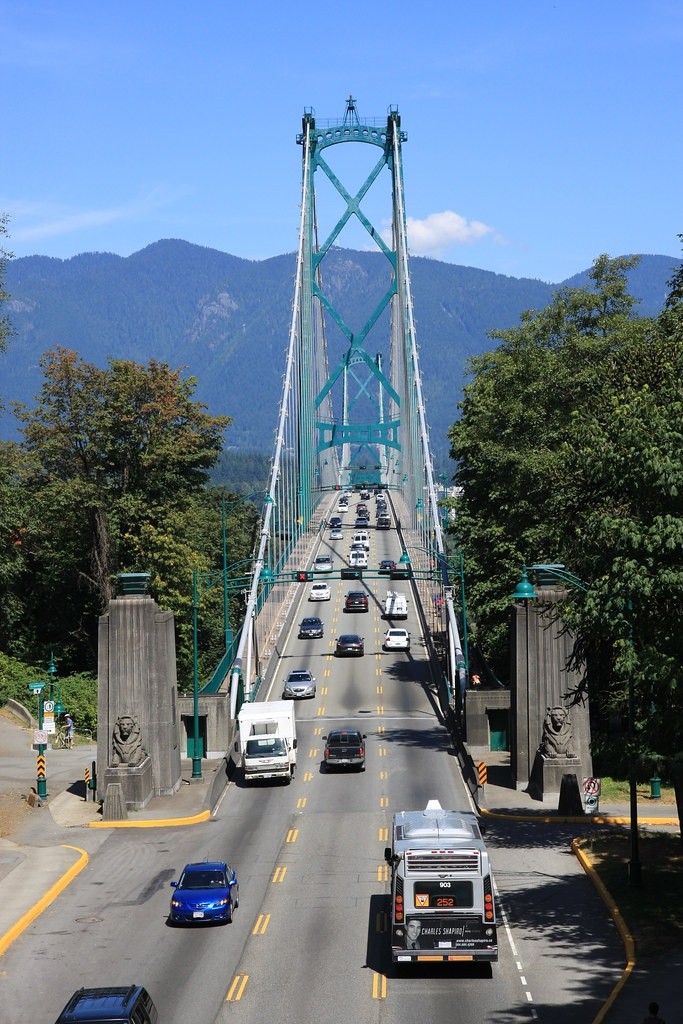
[412,942,415,949]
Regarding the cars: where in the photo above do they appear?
[309,583,332,601]
[282,668,318,700]
[313,554,334,572]
[330,489,394,529]
[298,617,325,639]
[334,634,366,657]
[378,560,397,574]
[328,529,344,540]
[167,860,240,929]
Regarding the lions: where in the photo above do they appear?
[538,705,577,760]
[110,713,149,768]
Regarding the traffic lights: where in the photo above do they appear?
[390,569,412,581]
[340,568,363,581]
[334,465,389,490]
[297,570,308,582]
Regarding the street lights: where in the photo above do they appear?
[35,683,67,799]
[222,487,275,651]
[512,558,644,923]
[398,545,471,691]
[191,556,274,779]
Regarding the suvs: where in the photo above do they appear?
[344,590,370,612]
[383,628,411,650]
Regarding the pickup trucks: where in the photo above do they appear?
[322,729,368,773]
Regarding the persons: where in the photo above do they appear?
[61,714,74,749]
[210,873,223,885]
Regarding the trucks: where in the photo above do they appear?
[351,530,372,550]
[347,550,369,569]
[231,700,299,785]
[383,800,506,966]
[382,591,409,619]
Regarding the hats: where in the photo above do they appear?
[64,713,70,717]
